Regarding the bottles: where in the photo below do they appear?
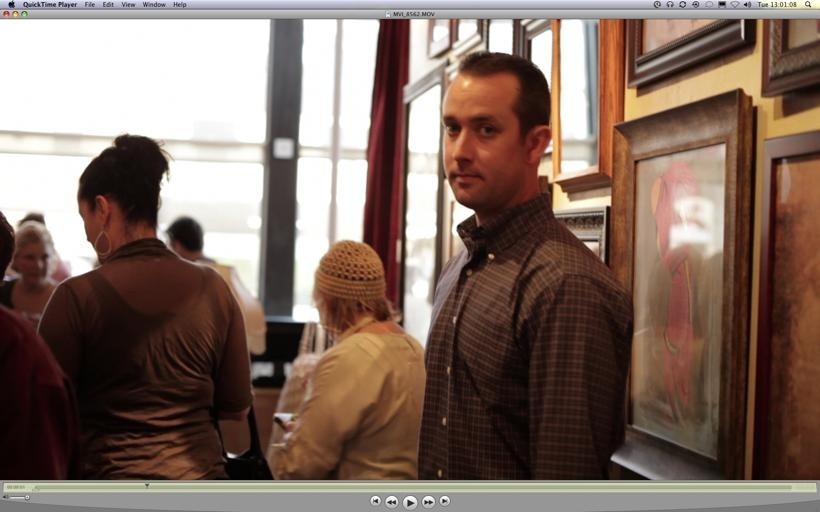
[315,240,387,300]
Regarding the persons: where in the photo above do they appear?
[38,135,255,480]
[166,216,267,355]
[268,241,428,481]
[0,212,83,480]
[416,52,635,480]
[13,213,70,281]
[1,220,65,330]
[628,160,716,451]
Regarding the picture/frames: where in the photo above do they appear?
[399,20,624,306]
[628,20,746,85]
[761,22,818,97]
[752,132,818,482]
[607,87,754,478]
